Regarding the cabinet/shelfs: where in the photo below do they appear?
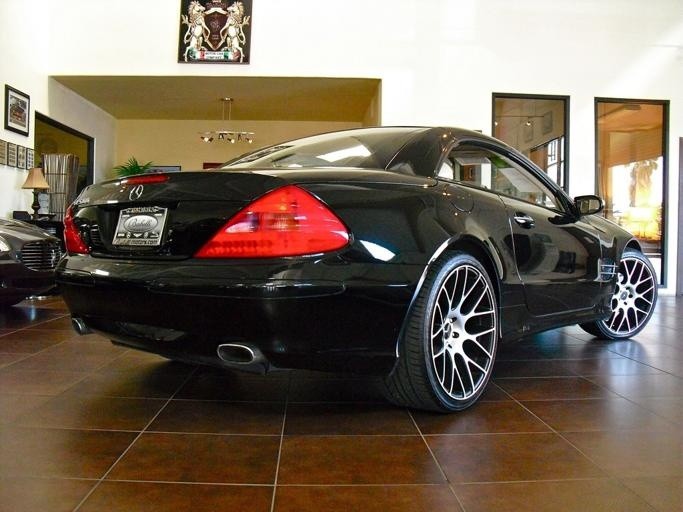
[18,218,65,254]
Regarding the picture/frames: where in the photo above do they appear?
[128,165,181,174]
[26,148,34,170]
[16,145,26,169]
[540,111,552,136]
[2,84,29,137]
[0,139,7,166]
[7,142,17,168]
[522,122,533,143]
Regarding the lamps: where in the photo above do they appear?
[197,97,255,144]
[21,167,50,218]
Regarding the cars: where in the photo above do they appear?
[56,124,664,411]
[0,213,65,313]
